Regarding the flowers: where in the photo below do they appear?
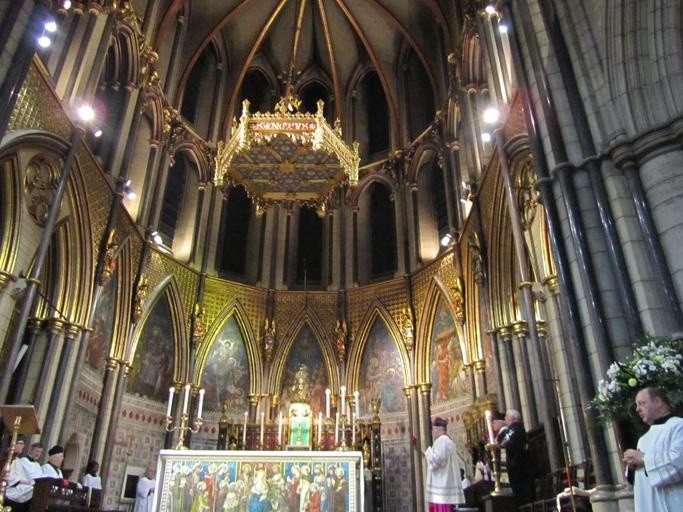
[583,330,683,423]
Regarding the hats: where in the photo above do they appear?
[506,408,520,419]
[434,417,447,427]
[49,446,64,456]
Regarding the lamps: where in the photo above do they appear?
[212,1,359,218]
[37,0,167,251]
[436,0,508,256]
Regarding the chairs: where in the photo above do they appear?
[519,461,591,512]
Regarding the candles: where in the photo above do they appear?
[162,383,362,449]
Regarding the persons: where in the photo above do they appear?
[2,442,44,511]
[452,468,479,512]
[482,411,511,488]
[80,461,103,490]
[495,408,532,512]
[424,416,467,511]
[168,459,350,512]
[621,386,682,512]
[133,463,157,512]
[39,444,83,506]
[12,438,27,461]
